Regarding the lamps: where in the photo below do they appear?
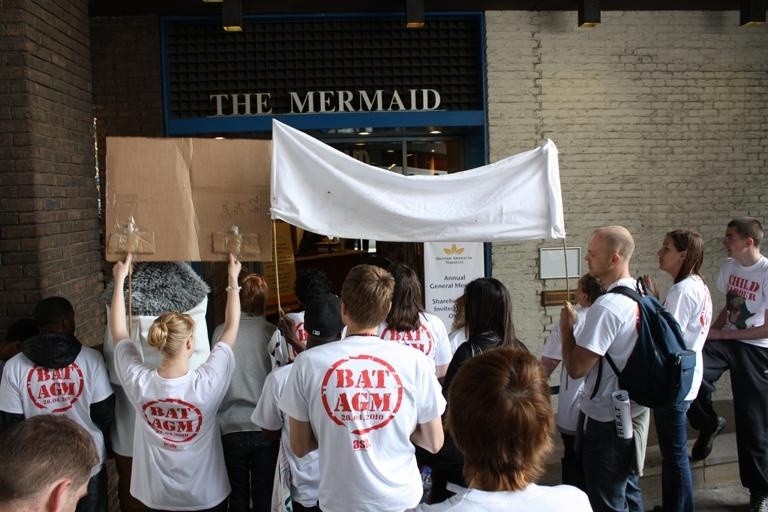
[406,1,425,29]
[221,1,245,33]
[740,6,765,28]
[578,4,600,29]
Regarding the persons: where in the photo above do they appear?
[643,229,713,512]
[560,225,650,512]
[1,252,608,512]
[685,217,768,512]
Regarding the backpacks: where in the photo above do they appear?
[601,284,696,410]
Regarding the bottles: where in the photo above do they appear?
[418,466,433,503]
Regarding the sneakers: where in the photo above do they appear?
[748,489,768,512]
[691,416,727,461]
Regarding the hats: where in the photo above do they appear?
[304,291,344,337]
